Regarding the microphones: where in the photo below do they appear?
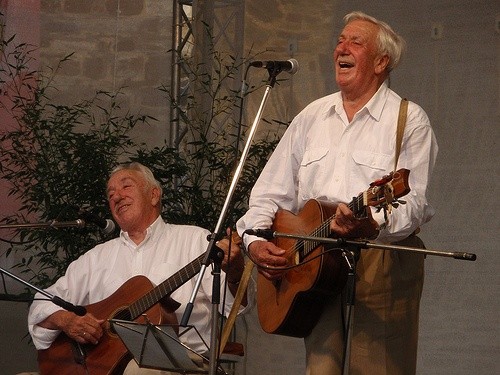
[52,296,87,316]
[250,58,300,74]
[71,204,116,235]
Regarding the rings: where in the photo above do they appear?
[81,333,86,337]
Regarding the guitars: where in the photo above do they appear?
[38,230,243,375]
[256,168,411,338]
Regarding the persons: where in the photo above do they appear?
[235,12,438,375]
[27,163,257,374]
